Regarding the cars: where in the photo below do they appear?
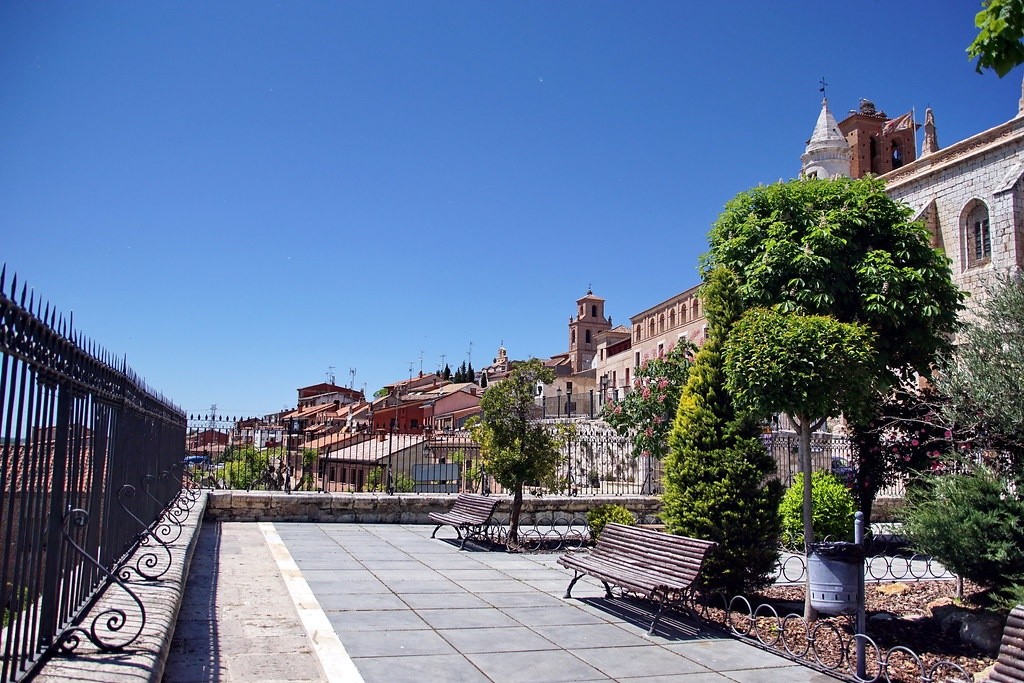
[199,477,209,487]
[208,465,215,470]
[215,463,225,470]
[182,482,215,491]
[831,456,860,493]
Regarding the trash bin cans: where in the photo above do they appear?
[803,539,862,614]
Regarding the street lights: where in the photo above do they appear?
[601,373,608,405]
[556,387,562,418]
[566,389,572,418]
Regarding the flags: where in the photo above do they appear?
[882,110,913,136]
[820,88,824,92]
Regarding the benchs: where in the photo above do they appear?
[429,493,505,551]
[556,521,718,637]
[987,605,1024,683]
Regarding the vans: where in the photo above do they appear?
[184,455,209,468]
